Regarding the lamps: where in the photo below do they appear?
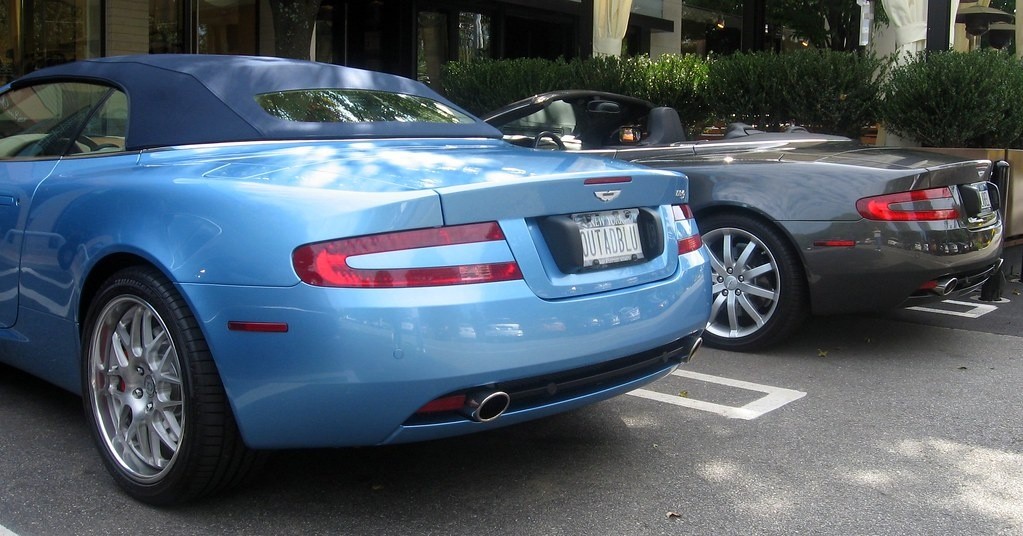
[802,38,809,47]
[955,4,1015,49]
[706,18,724,32]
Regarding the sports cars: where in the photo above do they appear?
[0,55,712,510]
[475,88,1008,354]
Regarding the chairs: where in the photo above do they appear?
[532,100,582,152]
[637,107,686,147]
